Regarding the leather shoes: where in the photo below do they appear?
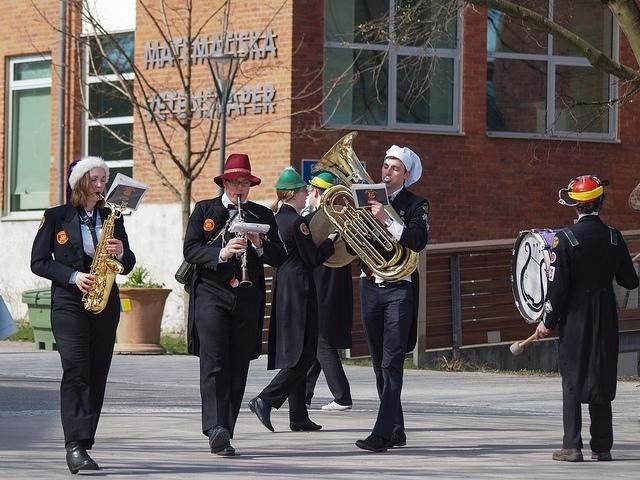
[290,419,321,431]
[209,426,235,455]
[248,397,274,430]
[66,442,99,474]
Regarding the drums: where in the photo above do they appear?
[509,227,558,325]
[310,204,358,268]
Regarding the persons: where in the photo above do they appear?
[534,174,640,463]
[330,145,430,452]
[304,173,354,413]
[249,168,337,432]
[28,156,136,474]
[184,154,288,457]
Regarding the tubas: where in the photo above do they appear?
[310,130,420,283]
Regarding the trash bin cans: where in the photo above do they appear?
[22,287,58,350]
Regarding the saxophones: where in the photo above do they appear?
[81,192,125,315]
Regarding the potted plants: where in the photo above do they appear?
[114,268,172,354]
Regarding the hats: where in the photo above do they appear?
[67,156,110,202]
[558,175,609,207]
[214,154,261,188]
[385,145,423,187]
[274,168,334,190]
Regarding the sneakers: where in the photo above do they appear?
[553,448,583,462]
[592,451,611,460]
[356,434,406,452]
[322,401,352,411]
